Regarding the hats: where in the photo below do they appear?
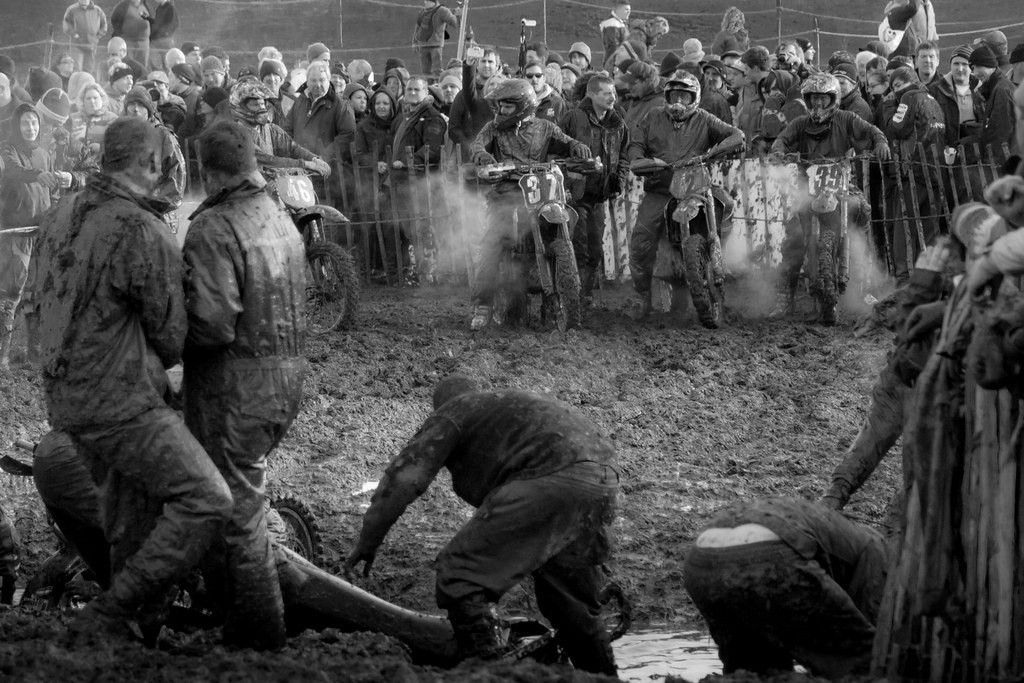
[426,58,463,109]
[0,42,229,123]
[948,32,1024,68]
[546,37,914,84]
[237,43,406,81]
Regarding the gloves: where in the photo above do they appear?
[480,153,496,166]
[769,151,785,164]
[570,143,592,160]
[872,142,892,160]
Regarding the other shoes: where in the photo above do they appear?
[768,292,795,322]
[221,615,287,653]
[470,304,493,331]
[630,292,651,321]
[67,597,154,647]
[439,614,499,670]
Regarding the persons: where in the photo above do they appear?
[33,111,313,648]
[345,375,618,678]
[0,1,1024,514]
[681,491,895,675]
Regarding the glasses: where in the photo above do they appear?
[526,73,544,79]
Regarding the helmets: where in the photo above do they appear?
[483,78,539,129]
[800,73,841,123]
[229,76,277,126]
[663,70,701,129]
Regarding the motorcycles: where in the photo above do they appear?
[473,157,586,330]
[254,162,360,337]
[134,496,326,655]
[635,141,744,330]
[775,152,893,326]
